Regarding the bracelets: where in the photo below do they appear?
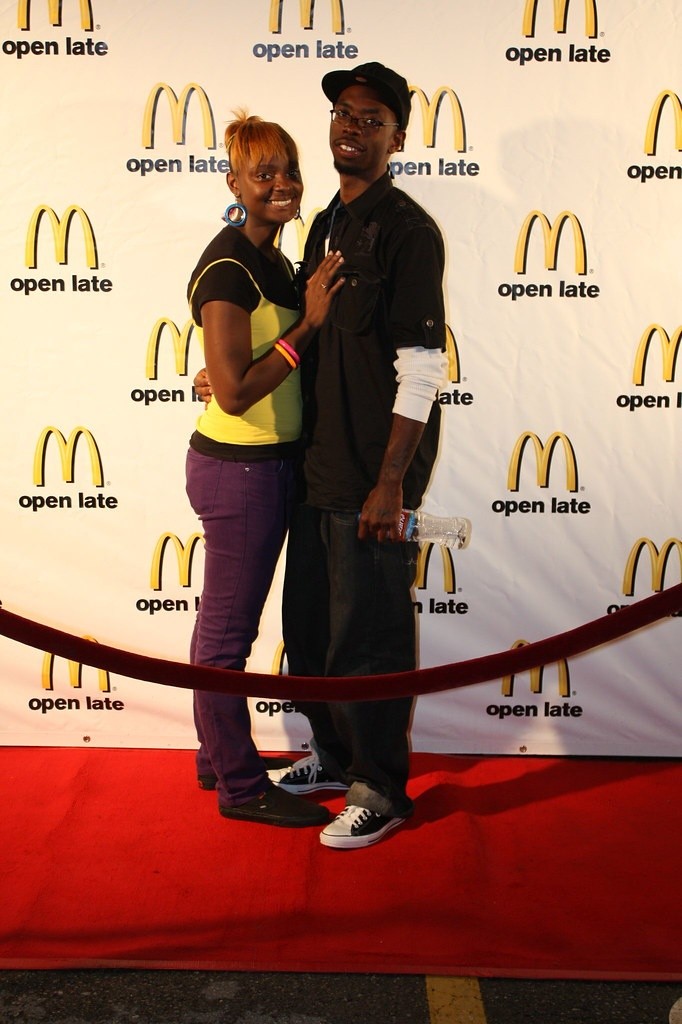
[274,338,300,369]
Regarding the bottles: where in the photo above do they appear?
[358,508,466,550]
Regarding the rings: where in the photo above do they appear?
[321,283,327,289]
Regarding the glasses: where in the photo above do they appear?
[330,108,400,134]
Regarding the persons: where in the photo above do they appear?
[184,108,346,827]
[194,61,449,848]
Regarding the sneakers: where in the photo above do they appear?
[278,748,351,795]
[219,782,330,828]
[197,755,296,790]
[320,796,412,848]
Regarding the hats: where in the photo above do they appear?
[321,61,411,126]
